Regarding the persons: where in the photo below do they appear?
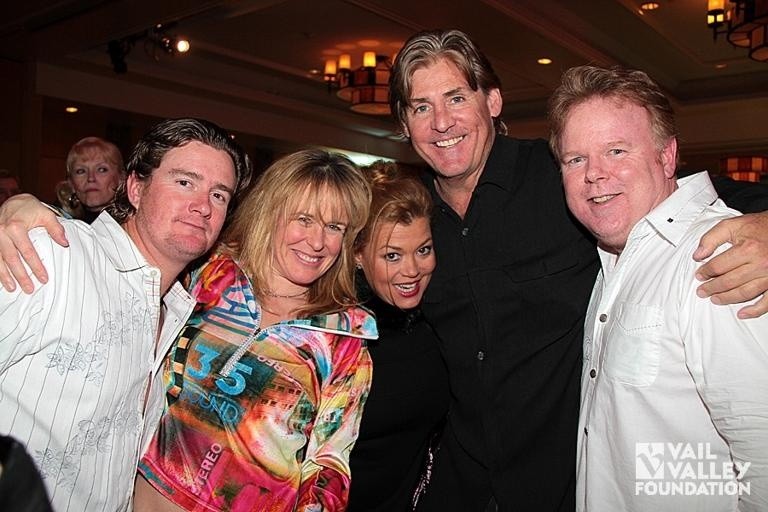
[347,155,447,511]
[380,27,768,512]
[546,62,767,511]
[0,113,257,511]
[0,146,374,512]
[57,134,126,228]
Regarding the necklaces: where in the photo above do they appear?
[266,283,312,298]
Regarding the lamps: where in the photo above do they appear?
[706,1,767,62]
[322,52,397,116]
[159,23,191,57]
